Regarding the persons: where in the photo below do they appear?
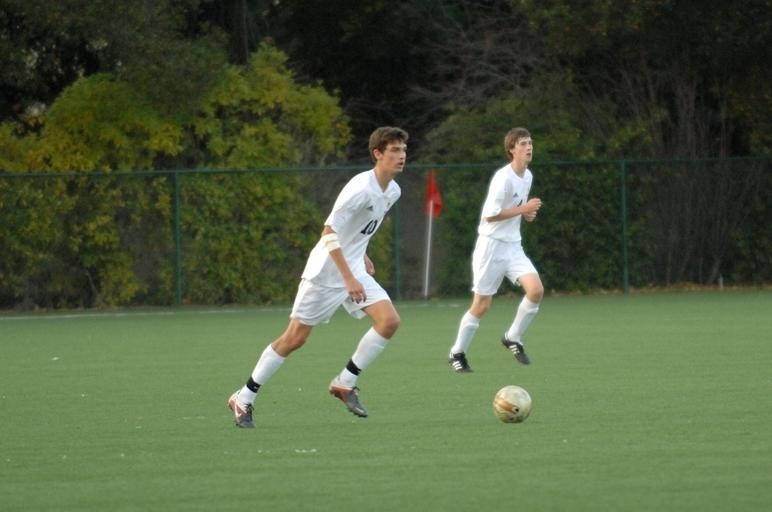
[226,124,411,430]
[445,126,545,372]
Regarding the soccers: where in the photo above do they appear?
[493,384,533,424]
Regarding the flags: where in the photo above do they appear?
[421,170,443,219]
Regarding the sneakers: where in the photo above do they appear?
[448,352,473,373]
[329,377,368,417]
[500,332,529,364]
[227,389,256,428]
